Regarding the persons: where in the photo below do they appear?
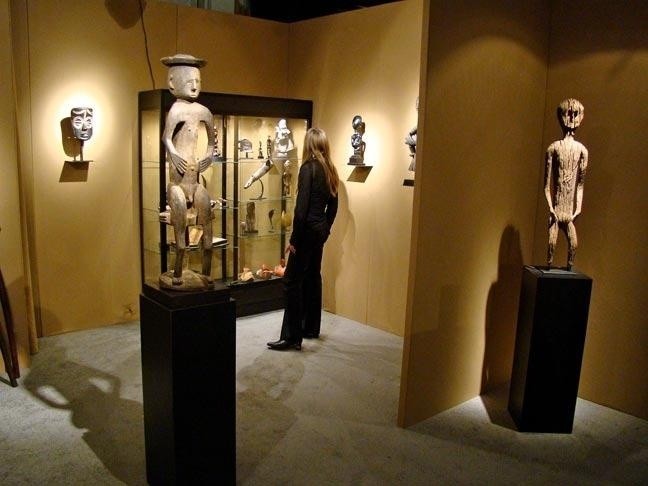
[160,53,217,288]
[266,128,339,352]
[70,106,93,141]
[544,98,589,273]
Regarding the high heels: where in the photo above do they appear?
[267,339,301,350]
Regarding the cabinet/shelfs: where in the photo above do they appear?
[138,88,313,318]
